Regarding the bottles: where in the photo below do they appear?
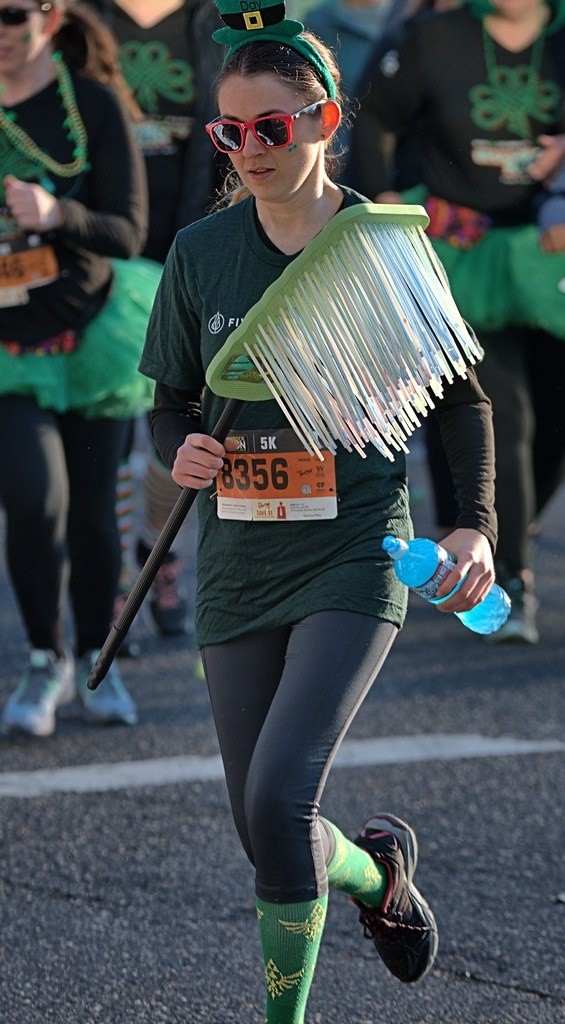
[383,536,512,635]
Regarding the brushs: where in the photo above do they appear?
[81,204,483,695]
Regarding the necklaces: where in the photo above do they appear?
[0,60,87,177]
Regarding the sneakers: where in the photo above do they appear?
[110,591,139,659]
[72,649,138,726]
[0,649,76,737]
[351,811,438,987]
[136,538,188,640]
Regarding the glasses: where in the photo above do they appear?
[0,3,53,26]
[205,99,328,153]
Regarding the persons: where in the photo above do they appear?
[138,0,499,1024]
[0,0,565,735]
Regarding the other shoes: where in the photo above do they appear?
[492,567,540,645]
